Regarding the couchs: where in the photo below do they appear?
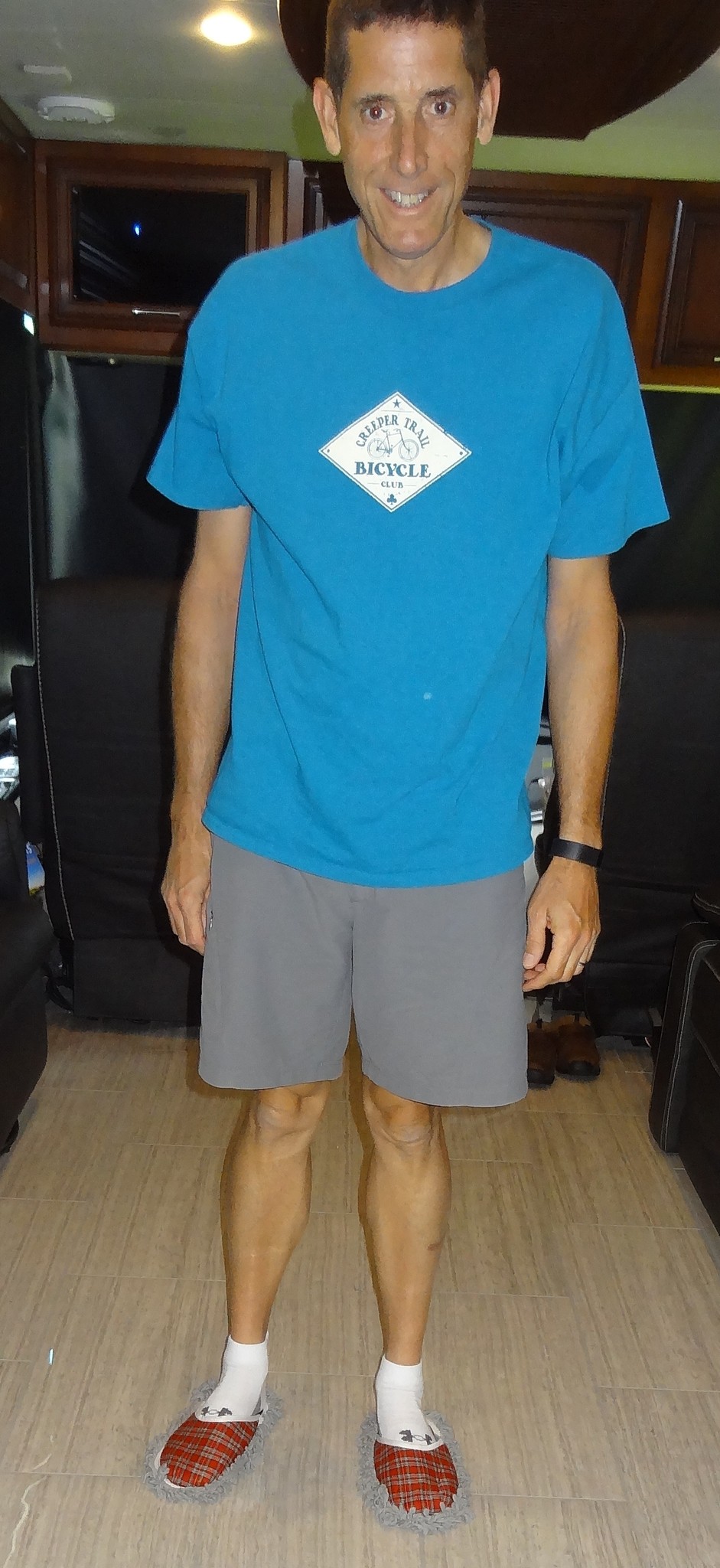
[10,572,202,1024]
[647,887,720,1234]
[535,601,720,1041]
[0,799,55,1155]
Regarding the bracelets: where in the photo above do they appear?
[545,837,601,868]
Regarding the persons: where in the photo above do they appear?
[141,1,683,1513]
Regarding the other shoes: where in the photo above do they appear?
[358,1410,470,1537]
[147,1381,284,1500]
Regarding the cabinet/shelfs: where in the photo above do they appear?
[36,139,720,396]
[0,95,37,316]
